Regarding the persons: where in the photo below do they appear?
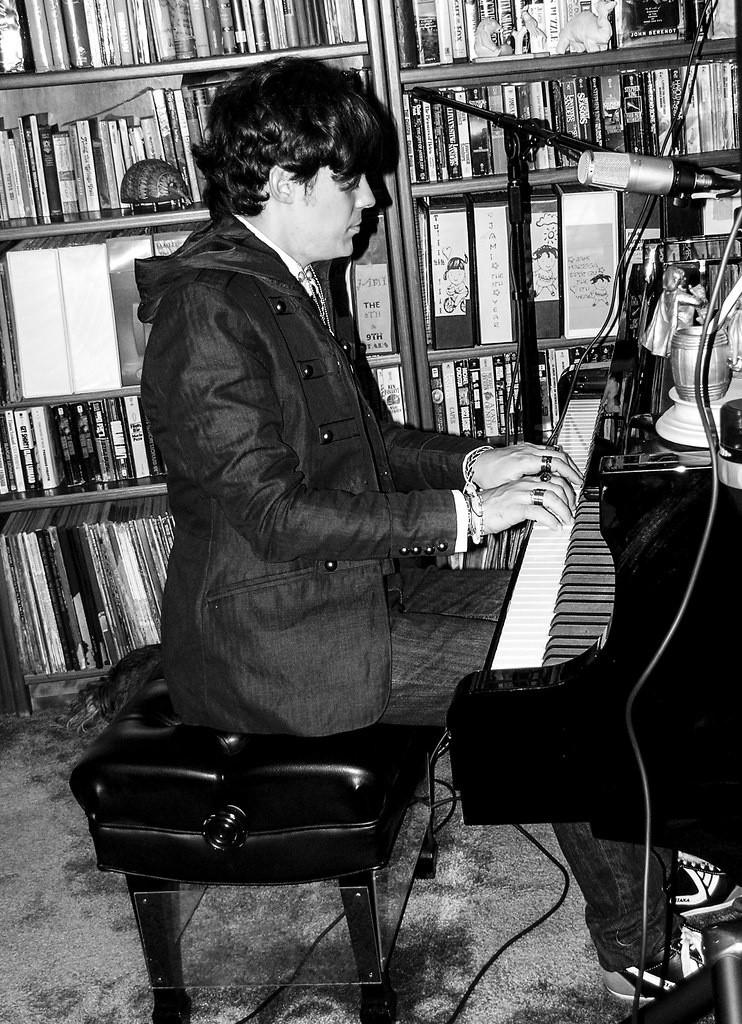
[133,53,740,1009]
[640,267,710,357]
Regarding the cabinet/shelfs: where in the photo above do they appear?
[0,2,418,728]
[377,1,740,563]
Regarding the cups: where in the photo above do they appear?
[671,326,732,402]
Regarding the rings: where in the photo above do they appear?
[541,455,547,472]
[546,456,552,472]
[531,488,545,505]
[539,471,552,482]
[553,444,560,451]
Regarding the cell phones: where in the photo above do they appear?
[600,452,680,475]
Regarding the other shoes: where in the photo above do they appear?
[667,849,741,918]
[600,921,705,1001]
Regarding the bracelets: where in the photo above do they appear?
[466,491,483,546]
[462,446,491,494]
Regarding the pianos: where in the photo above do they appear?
[448,237,741,1023]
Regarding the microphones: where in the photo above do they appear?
[575,148,742,198]
[411,85,525,133]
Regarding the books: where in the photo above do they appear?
[0,211,396,400]
[401,60,738,186]
[395,0,736,69]
[430,345,617,442]
[447,518,529,572]
[2,493,177,675]
[0,362,404,497]
[0,1,369,73]
[418,189,736,350]
[0,55,367,227]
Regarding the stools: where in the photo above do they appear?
[73,672,455,1023]
